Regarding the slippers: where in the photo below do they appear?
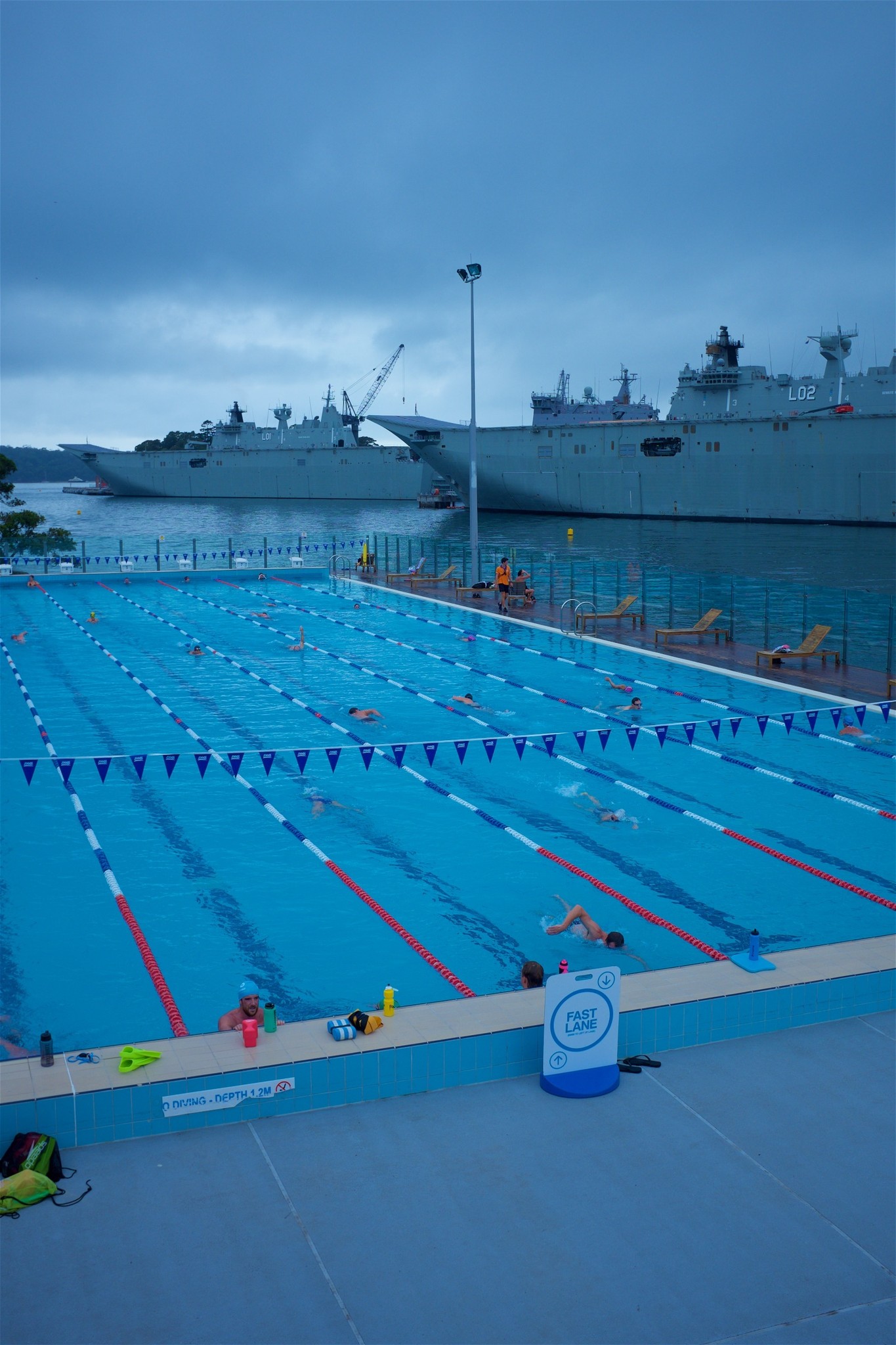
[617,1059,642,1074]
[623,1054,661,1068]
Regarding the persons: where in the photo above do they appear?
[594,696,647,716]
[604,675,634,693]
[85,612,99,623]
[218,981,285,1035]
[123,577,133,584]
[454,635,476,643]
[27,575,39,587]
[11,630,29,644]
[346,707,387,729]
[840,714,894,748]
[494,557,513,613]
[250,611,284,630]
[258,573,267,580]
[515,569,535,602]
[520,960,545,989]
[527,893,650,970]
[300,789,366,819]
[184,576,191,583]
[352,603,360,609]
[185,645,205,654]
[451,693,511,719]
[263,601,279,609]
[569,791,641,831]
[288,625,306,652]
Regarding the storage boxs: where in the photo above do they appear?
[509,581,525,595]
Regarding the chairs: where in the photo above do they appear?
[356,553,376,572]
[756,625,840,668]
[575,594,644,629]
[386,557,436,585]
[654,608,729,644]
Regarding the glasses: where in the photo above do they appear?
[77,1052,93,1062]
[635,703,643,706]
[611,815,618,822]
[196,648,201,650]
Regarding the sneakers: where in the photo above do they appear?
[497,601,502,611]
[503,607,508,612]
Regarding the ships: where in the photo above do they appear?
[61,315,896,529]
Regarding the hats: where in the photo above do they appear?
[194,645,201,651]
[90,612,95,618]
[614,808,626,820]
[468,635,476,641]
[843,715,854,726]
[238,980,260,1001]
[625,687,632,692]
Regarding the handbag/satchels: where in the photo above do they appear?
[0,1132,66,1182]
[0,1169,58,1214]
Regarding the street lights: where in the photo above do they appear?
[456,262,484,592]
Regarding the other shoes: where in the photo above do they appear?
[473,593,481,598]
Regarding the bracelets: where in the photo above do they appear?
[510,581,512,583]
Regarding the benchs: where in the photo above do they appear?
[455,588,500,601]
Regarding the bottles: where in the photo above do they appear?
[749,929,759,961]
[242,1019,259,1048]
[383,984,399,1016]
[39,1030,54,1068]
[263,1002,277,1032]
[559,959,568,974]
